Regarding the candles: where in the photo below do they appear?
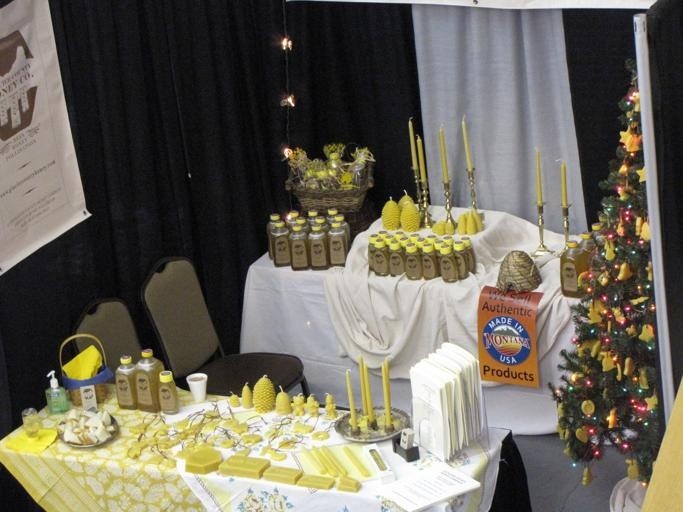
[534,147,543,206]
[416,135,426,180]
[462,114,473,171]
[409,117,418,169]
[560,161,568,207]
[346,356,392,429]
[439,124,448,183]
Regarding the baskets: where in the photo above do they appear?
[291,167,374,213]
[60,333,108,406]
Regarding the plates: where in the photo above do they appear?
[57,409,119,447]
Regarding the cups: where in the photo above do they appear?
[21,408,39,437]
[186,373,208,401]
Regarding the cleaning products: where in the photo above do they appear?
[45,369,70,415]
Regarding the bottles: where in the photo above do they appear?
[561,223,601,299]
[266,208,350,271]
[327,152,342,171]
[368,230,476,283]
[115,348,179,415]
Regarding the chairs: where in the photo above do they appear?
[140,256,311,402]
[70,299,142,384]
